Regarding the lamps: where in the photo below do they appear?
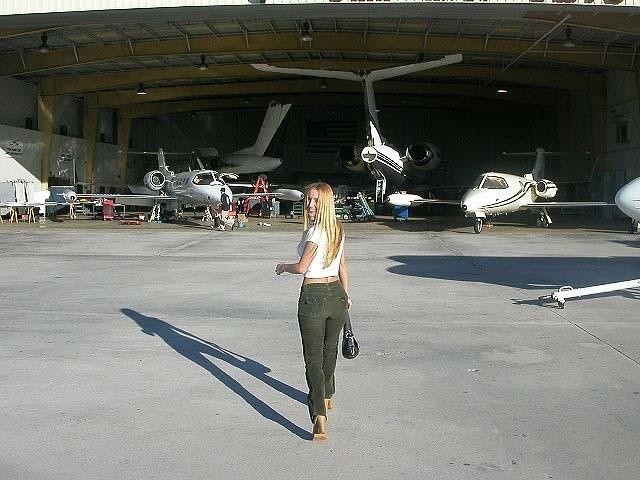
[299,16,314,42]
[36,28,50,55]
[197,54,208,71]
[495,78,510,95]
[560,23,576,49]
[135,81,147,95]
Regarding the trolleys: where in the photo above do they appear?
[291,202,304,218]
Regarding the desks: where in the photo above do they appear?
[0,198,128,226]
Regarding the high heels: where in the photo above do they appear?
[312,400,332,441]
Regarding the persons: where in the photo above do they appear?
[275,182,352,441]
[216,188,234,231]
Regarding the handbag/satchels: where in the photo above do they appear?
[342,328,359,359]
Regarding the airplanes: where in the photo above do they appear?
[414,148,617,234]
[56,148,323,225]
[364,190,461,222]
[117,101,293,178]
[250,53,603,218]
[613,177,640,221]
